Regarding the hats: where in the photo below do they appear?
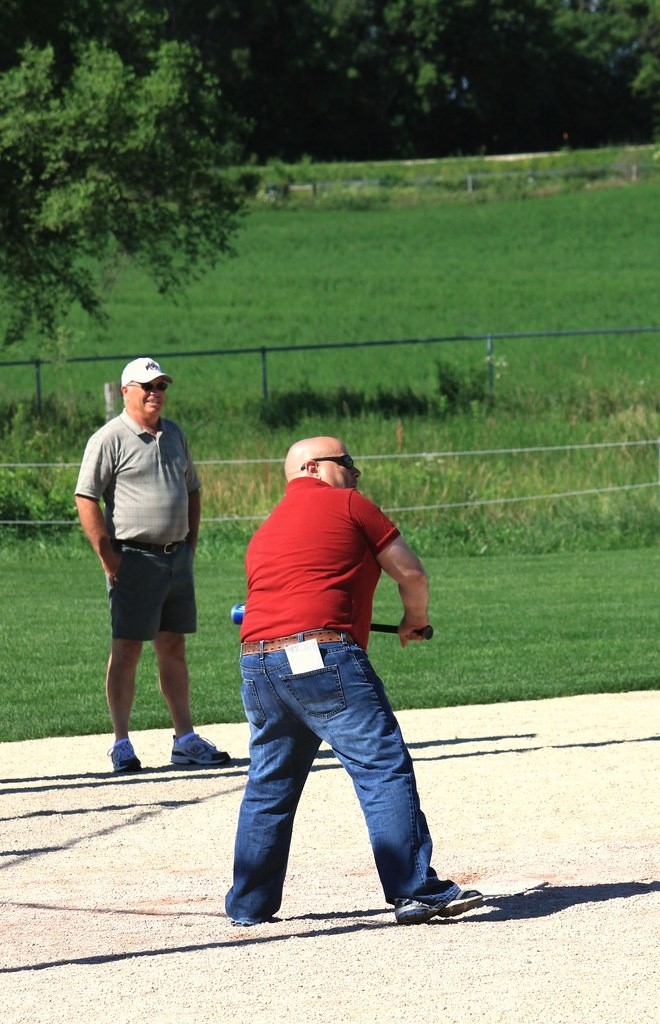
[121,358,173,388]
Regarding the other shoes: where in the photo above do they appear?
[394,890,483,924]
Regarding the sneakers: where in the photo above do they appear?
[171,733,230,764]
[106,740,141,772]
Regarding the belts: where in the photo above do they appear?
[242,629,354,656]
[110,537,188,555]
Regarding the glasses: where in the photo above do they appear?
[126,382,168,392]
[301,455,353,471]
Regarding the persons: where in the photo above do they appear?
[224,437,481,924]
[75,357,230,772]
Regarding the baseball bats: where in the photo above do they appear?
[231,603,434,641]
